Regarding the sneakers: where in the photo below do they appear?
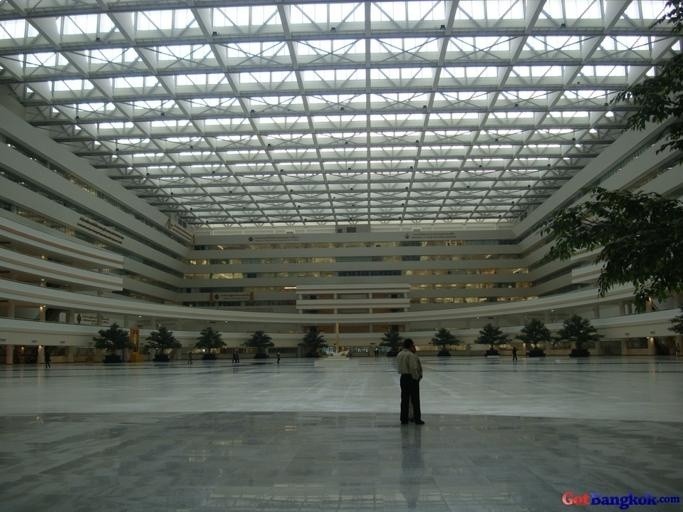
[400,419,425,426]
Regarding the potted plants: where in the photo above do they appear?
[297,327,328,357]
[554,313,603,358]
[429,329,459,357]
[242,331,274,358]
[193,327,226,360]
[378,327,406,357]
[93,323,135,362]
[514,318,554,357]
[475,323,512,357]
[145,326,181,362]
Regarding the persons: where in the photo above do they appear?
[669,339,675,358]
[276,352,281,363]
[77,313,82,324]
[44,350,51,368]
[408,344,422,422]
[396,338,425,426]
[186,350,240,365]
[511,346,518,361]
[675,343,680,358]
